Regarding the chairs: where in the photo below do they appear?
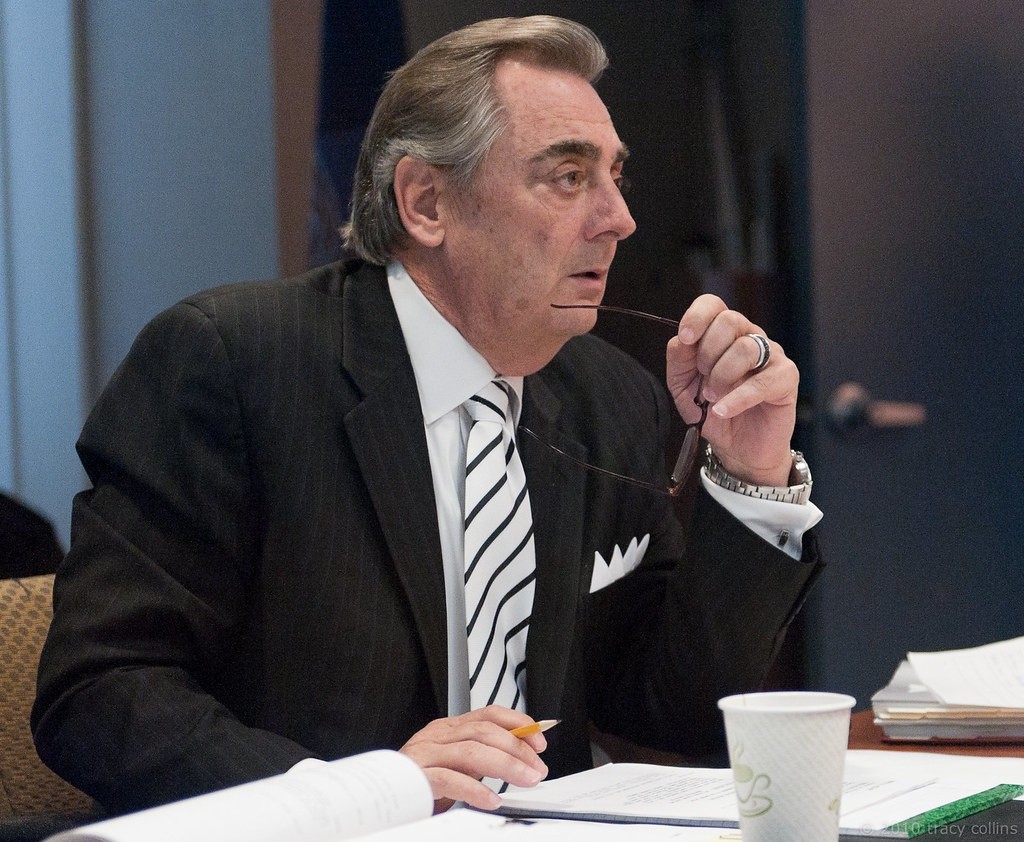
[0,574,112,842]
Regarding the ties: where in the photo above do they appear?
[462,380,537,799]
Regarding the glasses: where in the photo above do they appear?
[518,304,710,497]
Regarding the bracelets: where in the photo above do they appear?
[704,442,814,505]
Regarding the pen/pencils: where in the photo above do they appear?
[504,714,563,739]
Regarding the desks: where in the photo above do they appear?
[641,701,1023,769]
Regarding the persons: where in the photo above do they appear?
[29,14,830,819]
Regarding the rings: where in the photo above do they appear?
[745,333,772,377]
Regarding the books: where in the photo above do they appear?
[869,636,1024,744]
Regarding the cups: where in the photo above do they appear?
[718,690,857,842]
